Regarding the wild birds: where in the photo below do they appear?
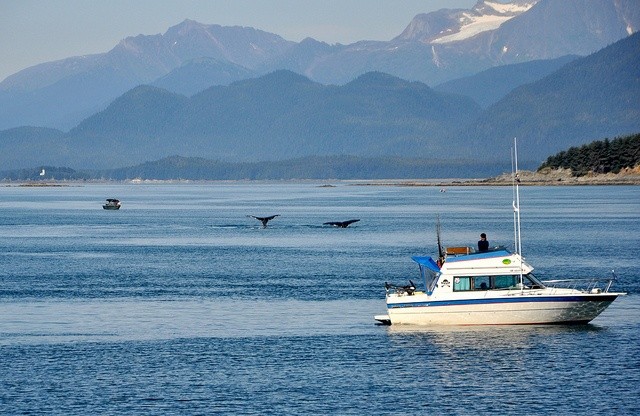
[246,213,281,227]
[323,219,360,228]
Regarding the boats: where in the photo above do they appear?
[102,199,121,210]
[373,136,628,326]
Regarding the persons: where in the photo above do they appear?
[478,233,489,252]
